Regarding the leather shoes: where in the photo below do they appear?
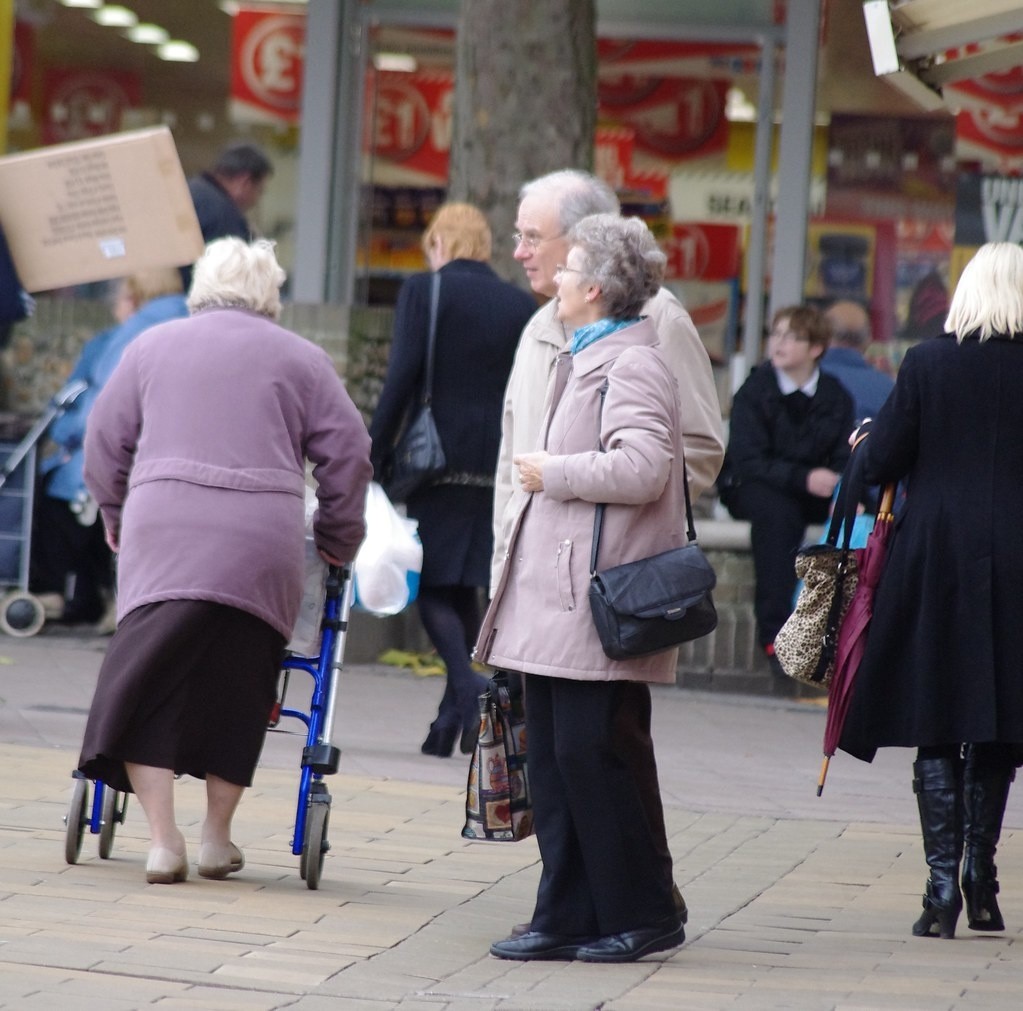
[490,920,686,963]
[511,880,688,936]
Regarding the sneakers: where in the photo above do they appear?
[97,602,118,635]
[32,592,64,620]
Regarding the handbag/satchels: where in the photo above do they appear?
[353,481,424,618]
[382,402,446,502]
[771,544,859,693]
[589,539,718,660]
[460,671,535,842]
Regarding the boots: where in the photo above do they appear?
[961,750,1017,931]
[912,758,966,938]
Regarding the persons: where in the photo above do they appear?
[23,282,140,634]
[83,264,190,414]
[845,240,1021,939]
[366,198,539,764]
[488,169,726,945]
[81,234,375,885]
[726,304,861,706]
[823,302,901,416]
[468,211,693,967]
[175,146,272,292]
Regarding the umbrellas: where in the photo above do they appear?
[816,433,898,799]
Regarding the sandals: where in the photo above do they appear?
[198,841,245,878]
[146,844,189,885]
